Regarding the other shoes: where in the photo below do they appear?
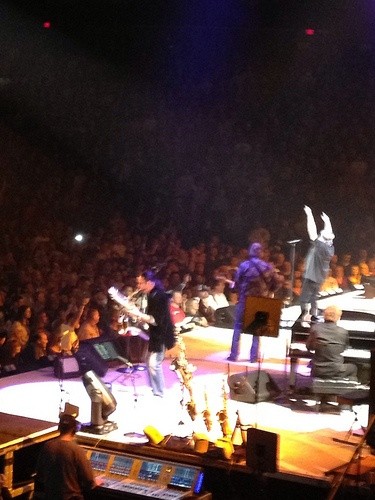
[227,356,237,362]
[311,315,320,321]
[298,309,308,318]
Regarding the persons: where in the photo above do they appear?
[34,414,106,500]
[306,305,358,412]
[0,184,375,377]
[123,269,175,400]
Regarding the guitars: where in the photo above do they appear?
[214,275,237,289]
[107,284,152,341]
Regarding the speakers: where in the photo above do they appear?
[228,369,283,403]
[54,353,82,378]
[214,305,240,328]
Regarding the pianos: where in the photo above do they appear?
[286,297,375,396]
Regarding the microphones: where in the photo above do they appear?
[287,238,305,247]
